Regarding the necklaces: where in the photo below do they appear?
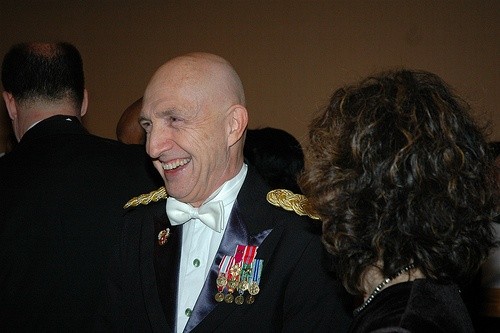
[353,262,422,316]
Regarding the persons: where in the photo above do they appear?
[477,141,500,289]
[92,52,350,333]
[297,68,500,333]
[0,38,148,333]
[242,127,304,188]
[116,98,147,144]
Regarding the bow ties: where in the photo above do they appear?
[166,196,224,233]
